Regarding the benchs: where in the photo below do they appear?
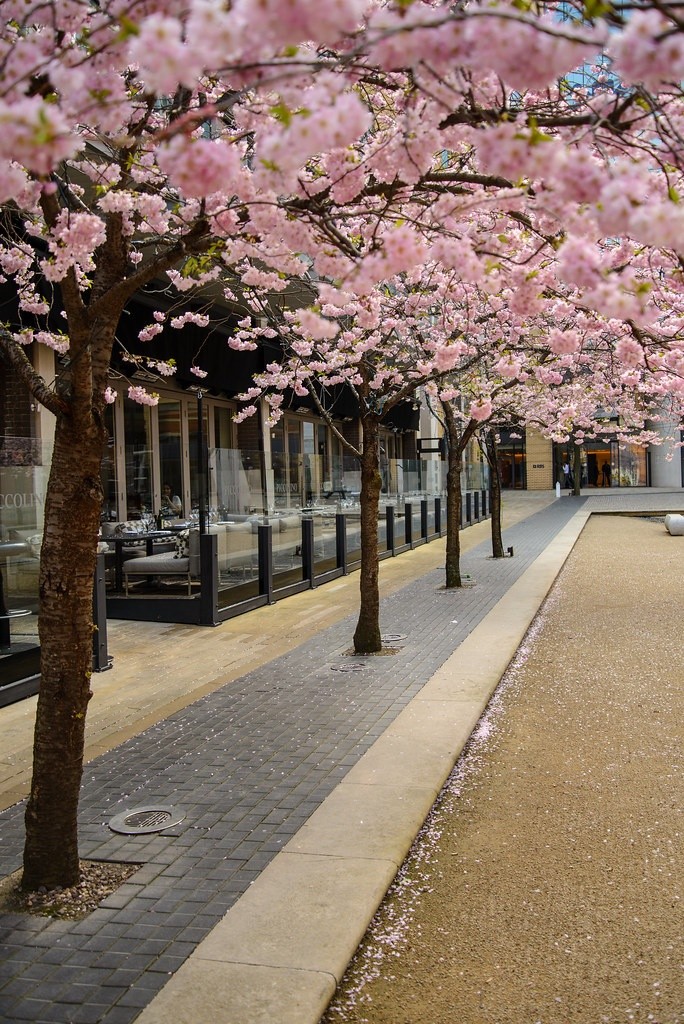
[9,495,450,597]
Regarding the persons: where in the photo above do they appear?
[161,485,181,519]
[592,461,599,487]
[562,461,571,484]
[602,461,611,488]
